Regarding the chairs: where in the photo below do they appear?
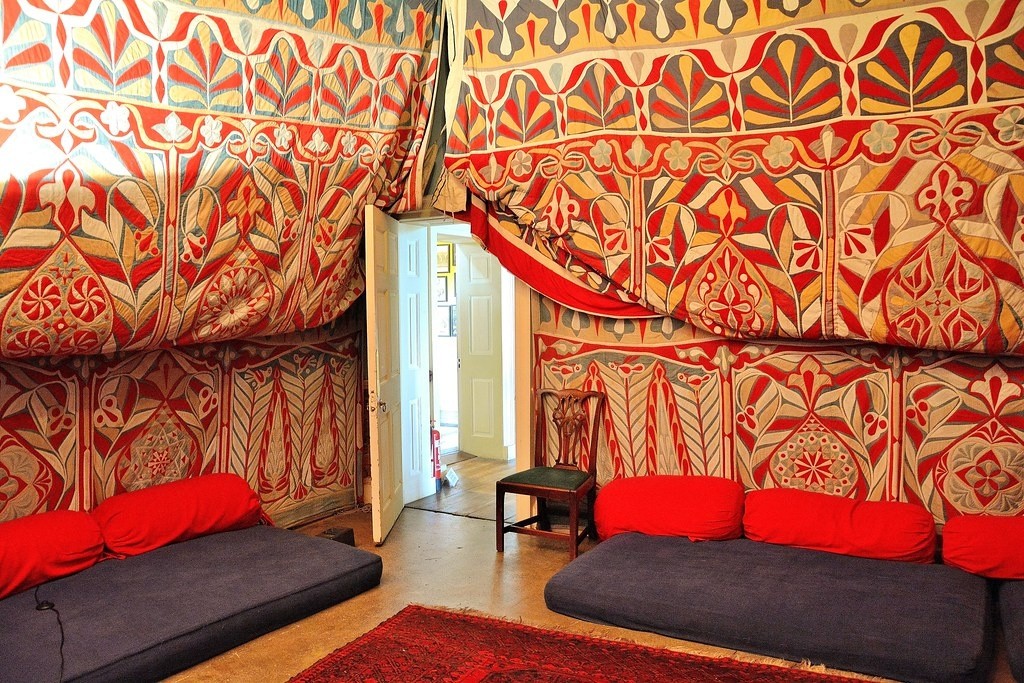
[496,388,605,562]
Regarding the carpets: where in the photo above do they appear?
[286,601,894,683]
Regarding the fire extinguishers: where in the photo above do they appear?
[430,420,442,479]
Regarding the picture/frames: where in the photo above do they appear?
[437,305,451,337]
[437,244,451,273]
[451,305,458,337]
[452,244,456,266]
[436,276,448,302]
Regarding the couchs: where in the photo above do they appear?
[0,473,383,683]
[543,475,1024,683]
